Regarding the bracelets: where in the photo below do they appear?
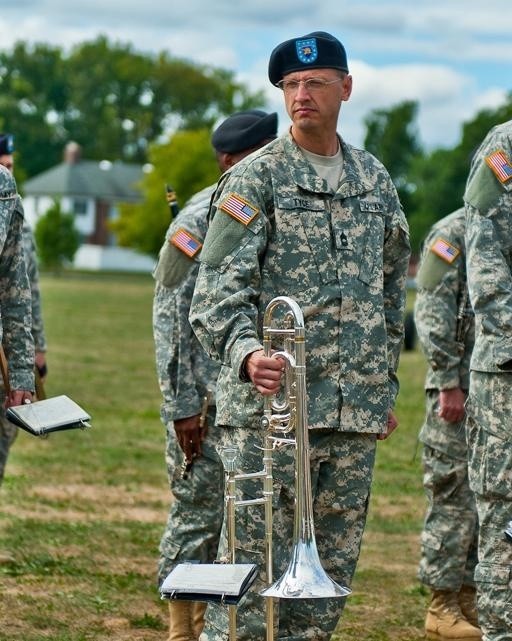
[35,364,47,379]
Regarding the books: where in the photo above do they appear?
[7,393,95,441]
[157,559,258,605]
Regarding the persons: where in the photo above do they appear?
[189,30,410,641]
[413,206,486,640]
[0,162,36,480]
[0,132,47,384]
[463,121,512,641]
[152,109,277,641]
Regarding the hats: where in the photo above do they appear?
[269,32,348,90]
[212,111,277,153]
[0,134,13,154]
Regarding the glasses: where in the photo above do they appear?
[276,78,342,92]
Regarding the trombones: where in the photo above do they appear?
[225,295,352,641]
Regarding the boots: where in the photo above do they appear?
[425,586,482,641]
[167,601,207,641]
